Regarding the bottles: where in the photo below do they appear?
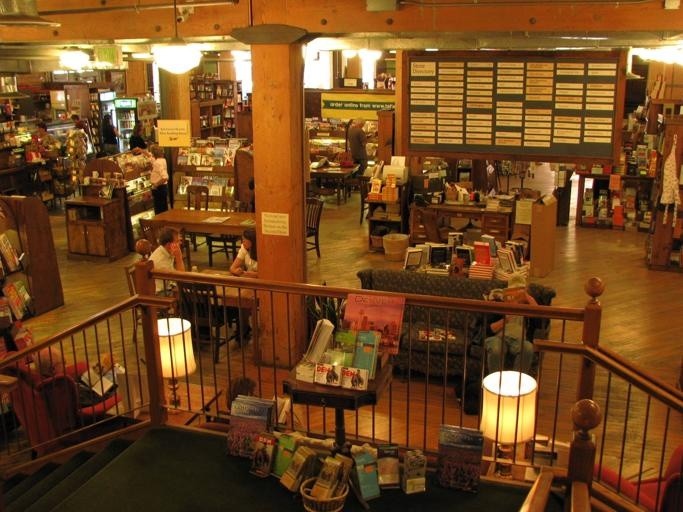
[191,265,198,284]
[118,110,134,119]
[120,130,132,139]
[120,121,134,129]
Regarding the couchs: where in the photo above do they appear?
[354,268,556,389]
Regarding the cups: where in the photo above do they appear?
[114,173,122,180]
[92,171,98,178]
[83,177,89,185]
[462,191,479,202]
[103,172,110,180]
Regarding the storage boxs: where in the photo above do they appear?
[529,193,557,277]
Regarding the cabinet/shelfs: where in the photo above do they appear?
[0,55,409,265]
[0,196,65,318]
[645,114,683,274]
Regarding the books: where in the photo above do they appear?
[0,233,35,364]
[403,232,531,281]
[175,80,256,226]
[80,357,125,399]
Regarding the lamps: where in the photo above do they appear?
[155,318,196,406]
[478,369,537,479]
[148,1,201,74]
[60,44,88,70]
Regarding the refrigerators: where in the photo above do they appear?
[89,91,118,153]
[114,98,136,151]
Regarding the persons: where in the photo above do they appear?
[0,95,169,214]
[469,284,538,374]
[135,227,185,318]
[229,228,256,342]
[349,119,367,191]
[33,348,125,445]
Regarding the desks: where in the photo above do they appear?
[282,353,393,443]
[408,198,512,244]
[195,269,261,366]
[134,379,223,426]
[573,172,653,230]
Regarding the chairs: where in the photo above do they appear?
[595,432,683,511]
[2,353,122,455]
[173,281,239,364]
[123,256,176,342]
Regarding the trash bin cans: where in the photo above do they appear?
[382,233,410,262]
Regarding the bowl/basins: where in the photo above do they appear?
[299,477,349,512]
